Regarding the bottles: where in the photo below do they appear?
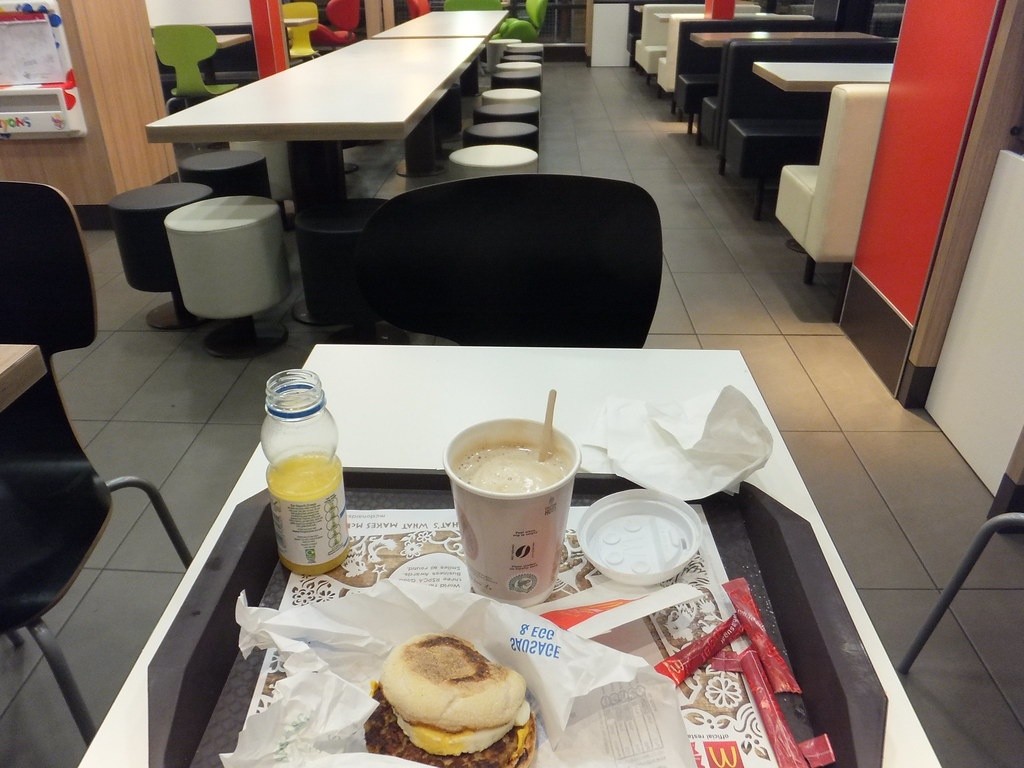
[260,369,350,574]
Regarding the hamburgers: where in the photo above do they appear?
[361,632,536,768]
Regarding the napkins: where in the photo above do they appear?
[580,378,775,503]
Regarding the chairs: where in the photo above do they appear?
[153,24,240,150]
[407,0,431,19]
[0,178,196,742]
[309,0,360,54]
[283,0,320,63]
[443,0,549,42]
[355,174,662,348]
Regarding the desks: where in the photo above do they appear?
[633,5,644,13]
[201,34,253,85]
[145,39,488,327]
[690,31,886,49]
[370,8,509,142]
[78,342,944,768]
[284,18,319,28]
[655,12,669,24]
[753,62,892,91]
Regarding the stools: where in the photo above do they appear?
[448,38,549,182]
[110,152,388,354]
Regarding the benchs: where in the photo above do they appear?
[626,0,904,320]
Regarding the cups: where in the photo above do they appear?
[443,418,582,609]
